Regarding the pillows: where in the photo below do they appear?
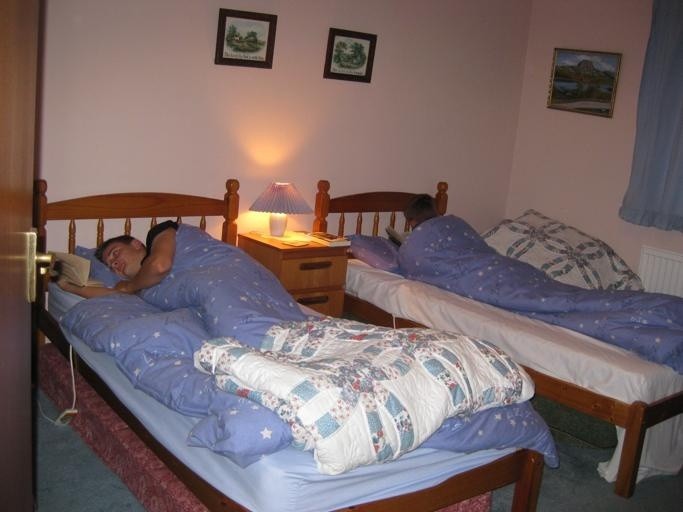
[74,245,120,289]
[345,233,396,271]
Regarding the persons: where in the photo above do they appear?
[53,221,178,299]
[404,194,440,231]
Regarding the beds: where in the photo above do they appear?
[313,179,683,498]
[34,178,560,510]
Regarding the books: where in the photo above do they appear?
[385,226,411,247]
[307,231,350,247]
[48,249,105,288]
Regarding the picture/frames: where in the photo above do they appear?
[322,28,377,84]
[213,8,277,70]
[546,47,622,119]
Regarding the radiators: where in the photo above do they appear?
[638,244,682,299]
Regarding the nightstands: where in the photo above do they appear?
[236,230,349,317]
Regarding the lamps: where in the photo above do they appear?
[248,181,312,237]
